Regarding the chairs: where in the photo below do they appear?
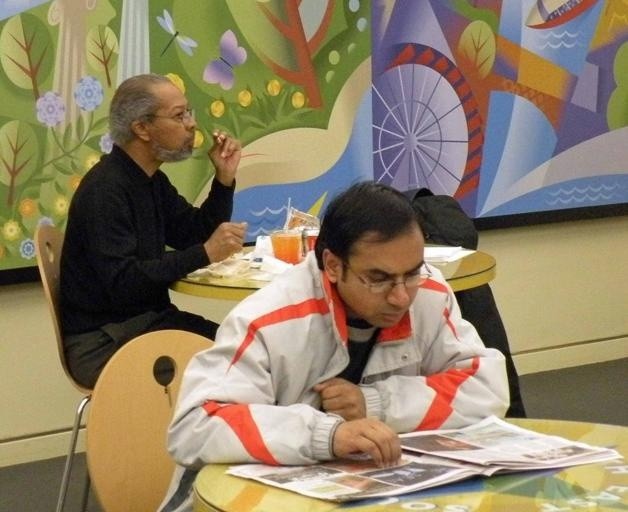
[34,224,94,512]
[86,330,216,512]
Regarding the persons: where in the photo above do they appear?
[58,72,242,390]
[401,187,527,418]
[156,180,510,512]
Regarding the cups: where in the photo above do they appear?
[255,236,271,256]
[303,229,320,251]
[270,230,303,264]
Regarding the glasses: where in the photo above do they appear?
[139,109,193,122]
[347,260,433,293]
